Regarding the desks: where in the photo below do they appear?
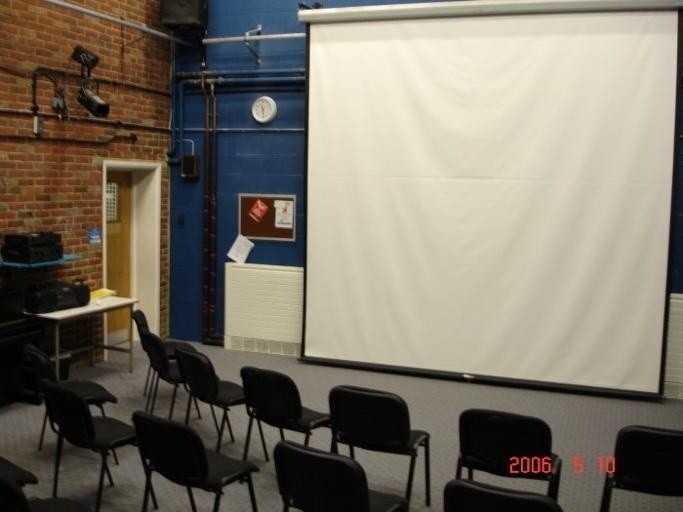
[24,294,139,382]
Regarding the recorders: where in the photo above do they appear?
[25,283,91,314]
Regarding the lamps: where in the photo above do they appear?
[76,76,112,119]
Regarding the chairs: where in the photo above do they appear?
[598,420,681,510]
[172,347,271,463]
[325,383,433,508]
[440,476,565,511]
[237,363,358,464]
[0,307,681,511]
[271,439,412,511]
[127,309,203,426]
[0,369,161,510]
[452,406,566,500]
[128,408,261,512]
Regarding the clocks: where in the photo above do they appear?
[249,96,282,130]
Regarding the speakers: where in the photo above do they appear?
[159,0,209,38]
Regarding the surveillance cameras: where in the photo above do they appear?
[76,88,110,118]
